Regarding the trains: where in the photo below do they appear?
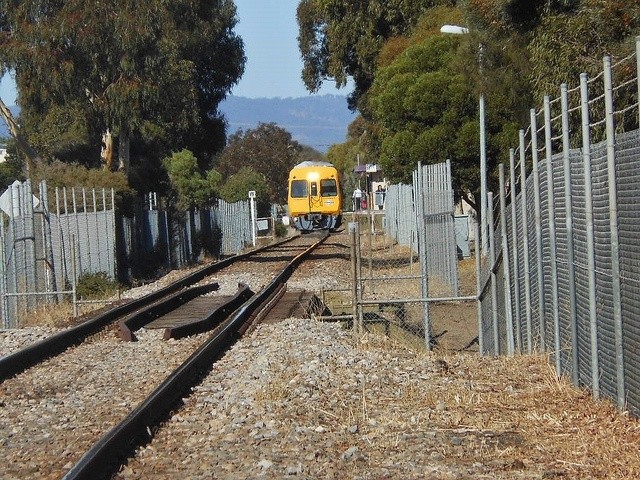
[287,160,343,231]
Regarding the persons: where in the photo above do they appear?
[376,185,385,210]
[351,184,362,210]
